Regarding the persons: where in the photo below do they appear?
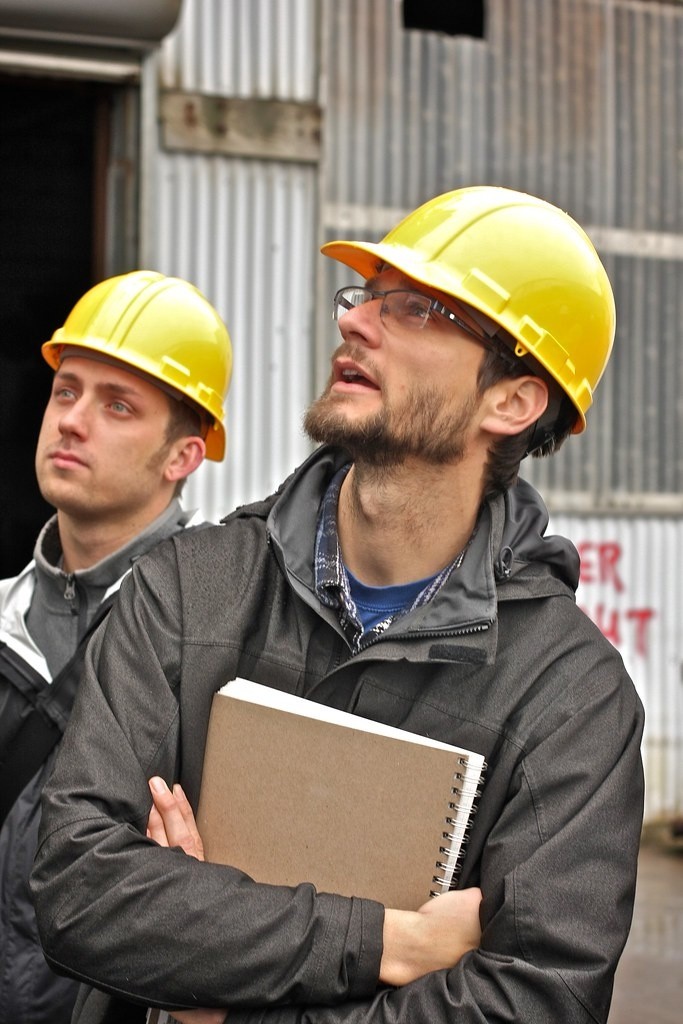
[30,183,646,1024]
[0,268,238,1024]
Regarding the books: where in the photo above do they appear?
[181,677,486,911]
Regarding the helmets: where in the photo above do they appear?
[41,270,234,462]
[320,187,615,434]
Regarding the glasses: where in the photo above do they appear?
[332,285,520,370]
